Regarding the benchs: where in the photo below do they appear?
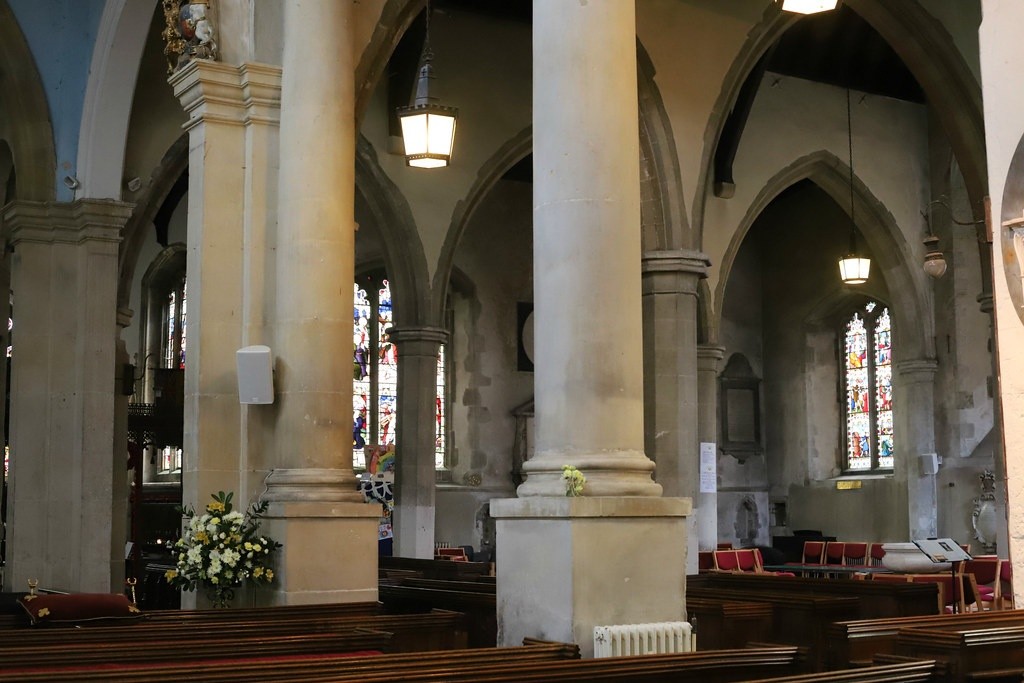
[0,549,1024,683]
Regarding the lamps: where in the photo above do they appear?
[921,196,993,278]
[773,0,843,15]
[837,5,872,286]
[398,0,460,169]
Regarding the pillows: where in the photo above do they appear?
[16,591,144,626]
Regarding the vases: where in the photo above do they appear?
[205,574,247,607]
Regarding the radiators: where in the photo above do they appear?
[594,613,691,657]
[435,541,450,548]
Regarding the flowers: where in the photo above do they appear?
[557,464,587,497]
[157,490,281,595]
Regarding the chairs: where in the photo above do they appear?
[438,547,464,556]
[700,540,1014,606]
